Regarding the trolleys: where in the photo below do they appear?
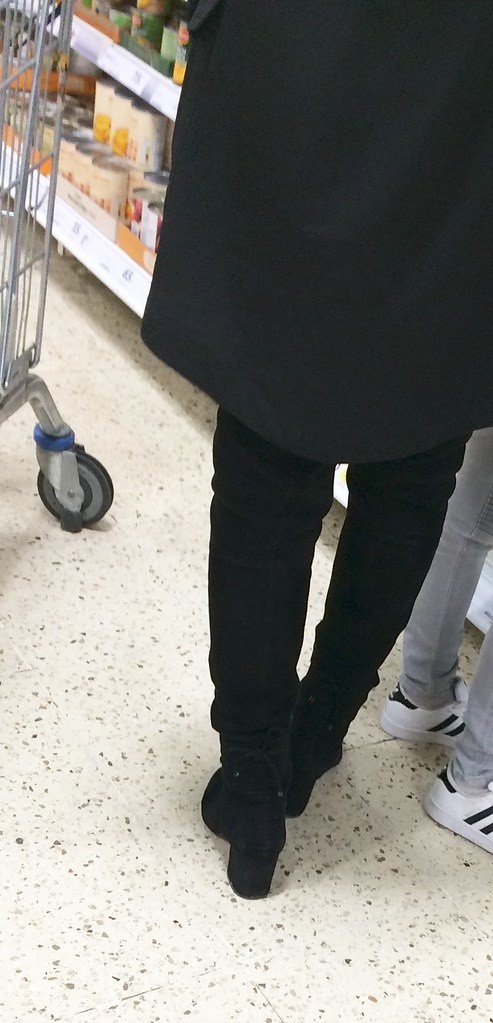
[0,1,114,534]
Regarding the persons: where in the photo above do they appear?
[140,0,493,900]
[379,428,493,852]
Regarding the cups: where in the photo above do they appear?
[173,18,190,85]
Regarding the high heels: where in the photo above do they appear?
[200,765,287,900]
[286,790,312,817]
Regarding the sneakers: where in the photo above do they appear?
[423,753,493,854]
[380,670,471,749]
